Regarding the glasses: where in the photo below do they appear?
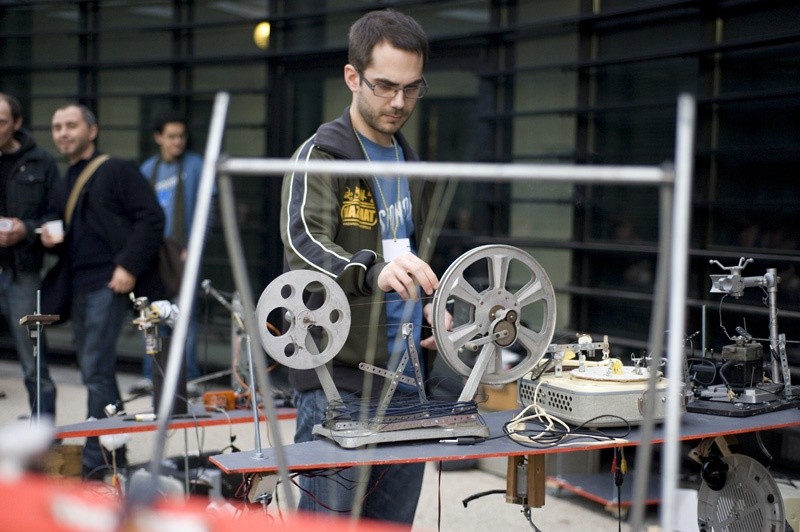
[355,69,428,99]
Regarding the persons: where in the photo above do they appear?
[139,111,219,390]
[32,102,165,482]
[280,8,453,522]
[0,93,64,423]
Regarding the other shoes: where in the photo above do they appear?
[127,379,155,396]
[185,380,206,398]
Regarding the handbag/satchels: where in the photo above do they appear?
[37,260,77,327]
[148,234,187,300]
